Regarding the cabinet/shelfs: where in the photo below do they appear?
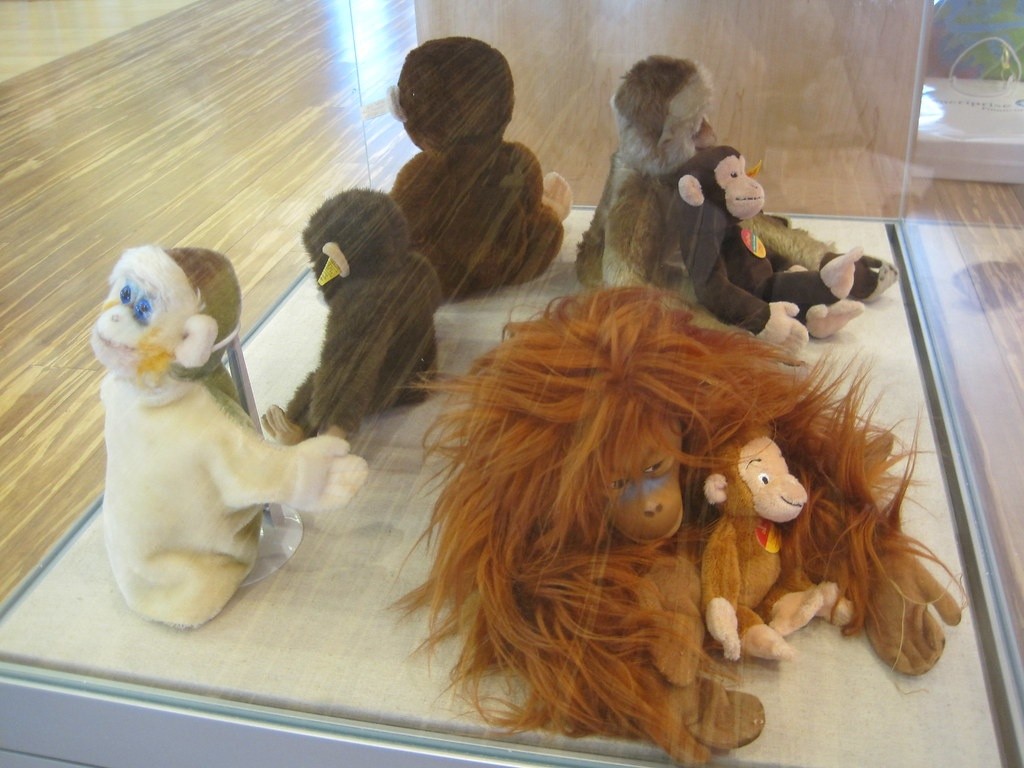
[0,0,1024,768]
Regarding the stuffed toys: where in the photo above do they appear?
[257,189,442,448]
[91,246,370,626]
[384,35,572,301]
[380,286,961,768]
[574,55,897,352]
